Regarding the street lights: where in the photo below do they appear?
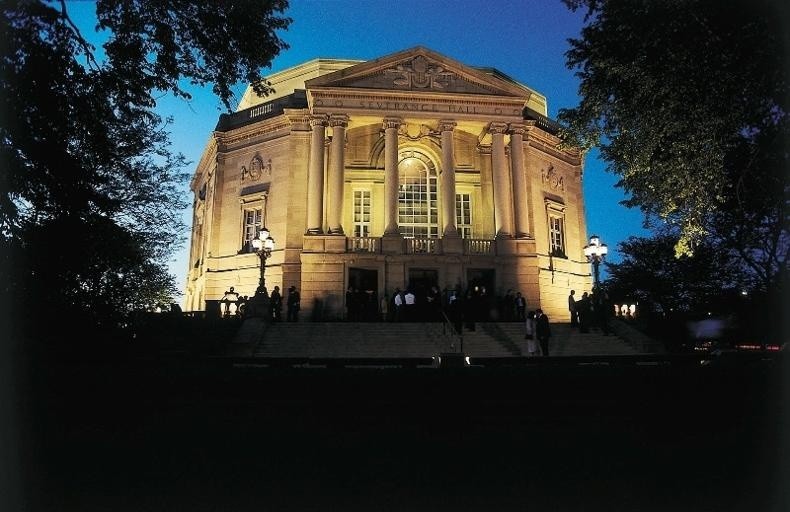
[251,227,275,288]
[582,237,608,282]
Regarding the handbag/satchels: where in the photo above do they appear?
[525,334,533,340]
